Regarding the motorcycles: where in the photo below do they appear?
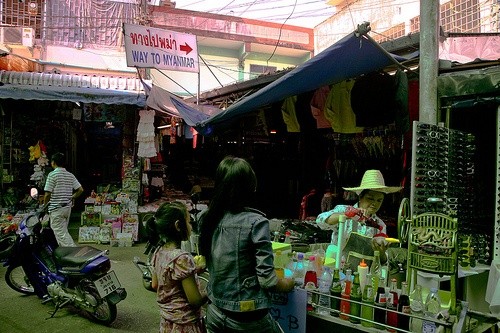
[4,186,127,325]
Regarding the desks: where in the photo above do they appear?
[137,196,193,238]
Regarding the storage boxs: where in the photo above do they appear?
[270,240,293,255]
[78,211,139,243]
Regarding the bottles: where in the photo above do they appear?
[273,232,279,242]
[345,206,383,231]
[284,232,291,243]
[286,251,441,333]
[273,251,284,279]
[91,190,96,197]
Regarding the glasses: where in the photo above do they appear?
[428,131,441,139]
[456,229,490,267]
[427,169,441,178]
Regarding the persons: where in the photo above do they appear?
[44,153,83,247]
[198,158,295,333]
[146,203,209,333]
[316,170,402,251]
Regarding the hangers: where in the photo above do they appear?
[321,70,391,86]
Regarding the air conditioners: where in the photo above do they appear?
[1,26,35,47]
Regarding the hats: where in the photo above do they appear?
[344,170,403,194]
